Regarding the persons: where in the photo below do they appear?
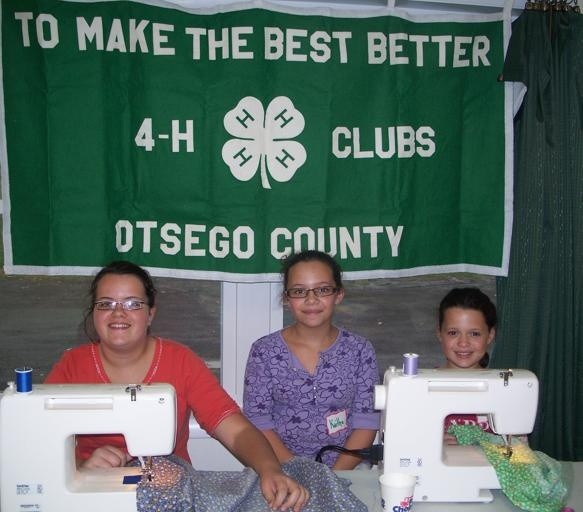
[45,260,309,512]
[435,286,511,445]
[243,248,381,480]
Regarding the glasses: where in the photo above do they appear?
[92,299,151,312]
[284,285,338,299]
